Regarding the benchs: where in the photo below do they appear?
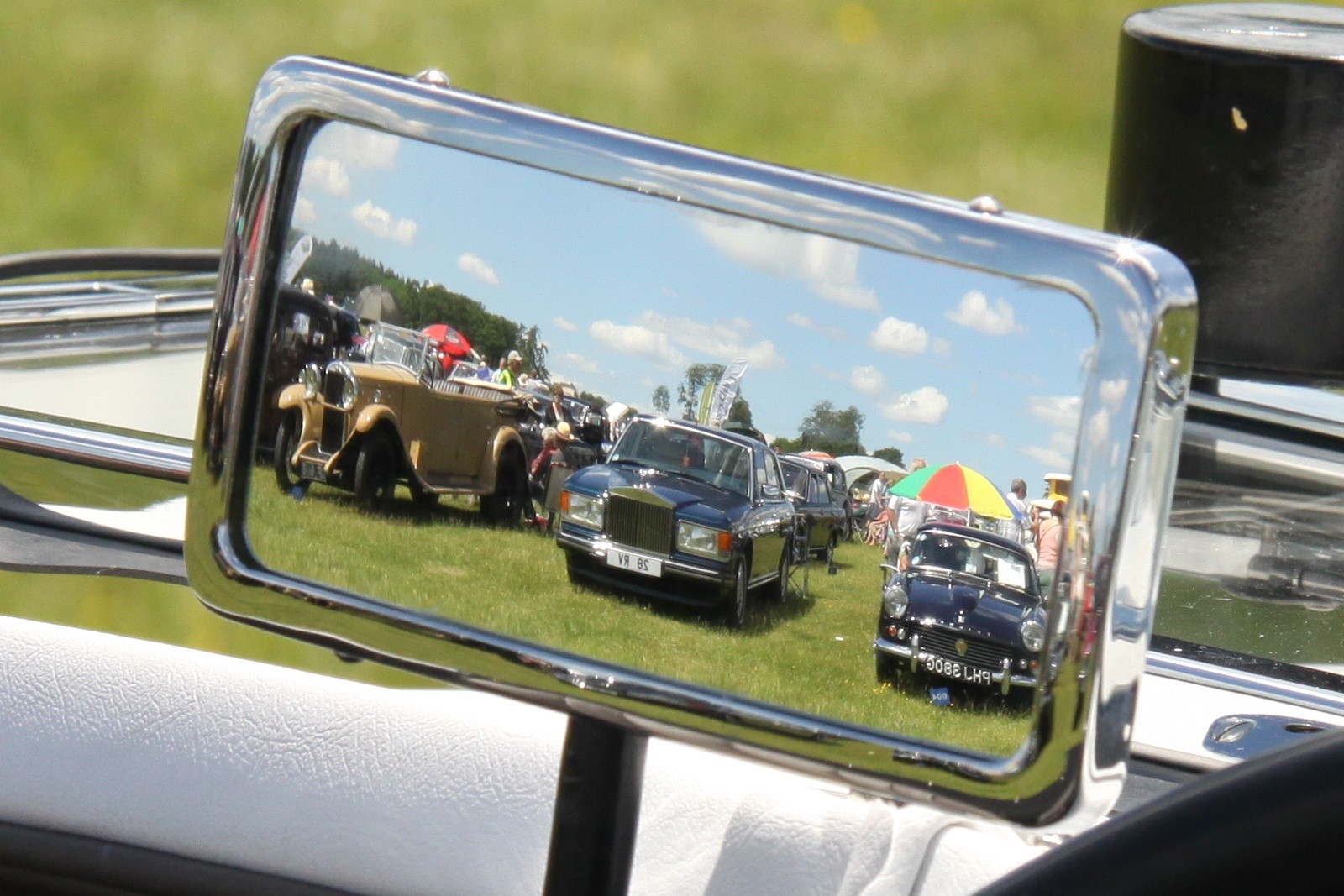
[425,375,507,405]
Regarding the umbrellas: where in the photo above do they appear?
[352,283,404,327]
[416,323,472,386]
[798,448,836,462]
[887,460,1021,527]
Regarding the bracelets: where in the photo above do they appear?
[892,528,899,535]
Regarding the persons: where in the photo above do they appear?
[860,458,927,567]
[292,279,359,346]
[475,349,576,529]
[996,478,1083,602]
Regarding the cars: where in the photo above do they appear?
[254,278,865,626]
[873,525,1050,712]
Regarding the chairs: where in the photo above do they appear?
[648,437,681,468]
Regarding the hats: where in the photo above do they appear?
[554,421,574,441]
[508,350,522,361]
